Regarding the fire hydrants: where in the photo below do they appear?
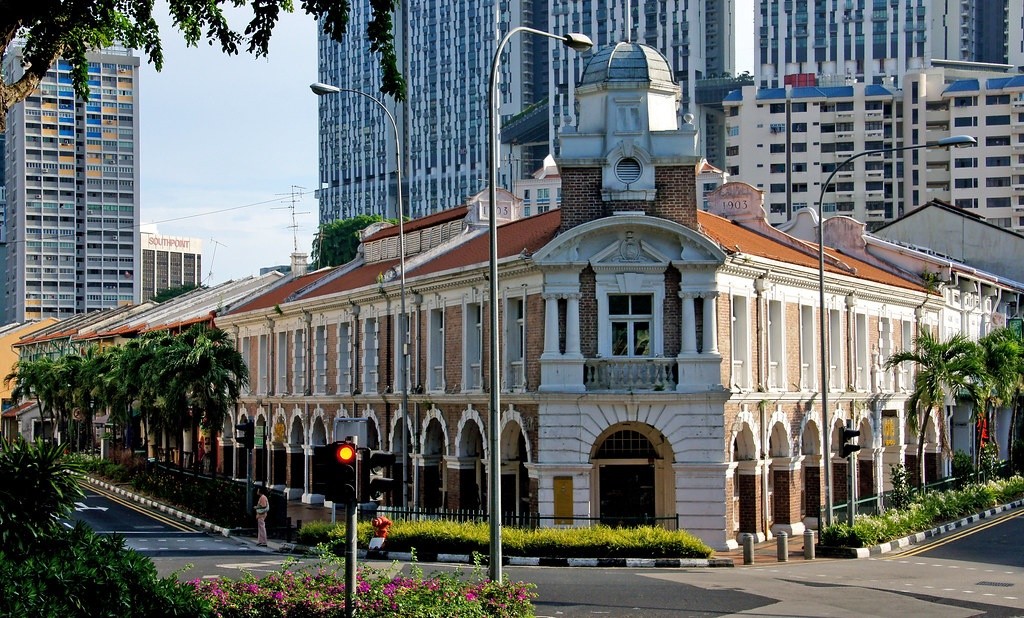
[372,516,392,548]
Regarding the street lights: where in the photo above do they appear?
[487,27,593,590]
[309,82,403,515]
[819,133,976,527]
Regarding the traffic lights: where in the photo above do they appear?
[328,442,355,506]
[312,444,332,503]
[235,423,254,449]
[363,448,396,502]
[839,426,861,459]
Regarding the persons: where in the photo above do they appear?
[254,489,270,547]
